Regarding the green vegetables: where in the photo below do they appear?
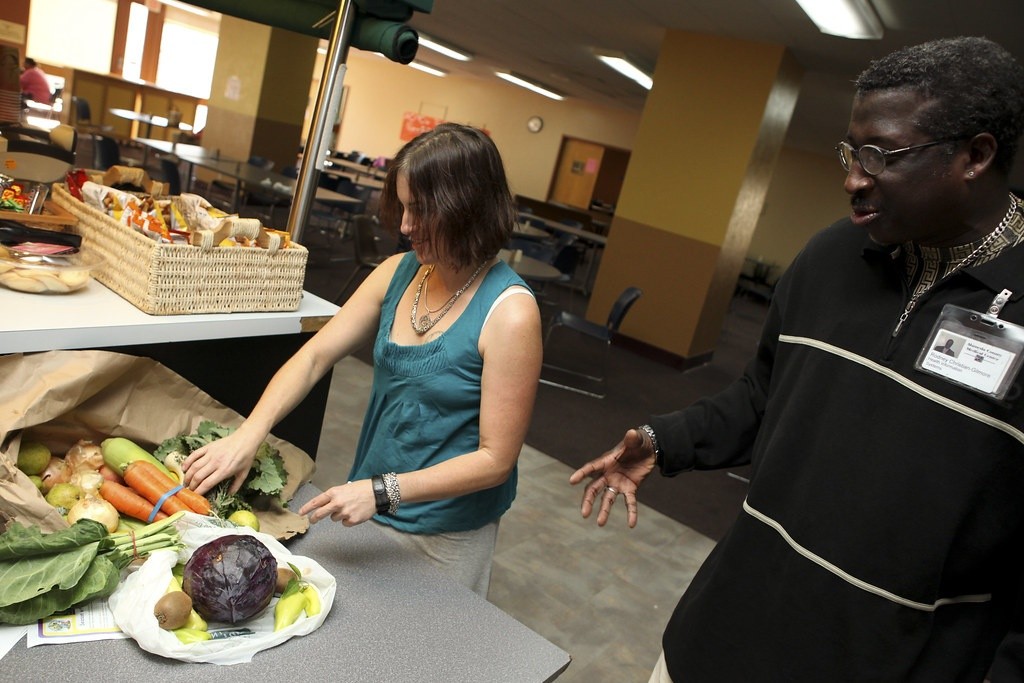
[0,511,184,623]
[152,420,290,520]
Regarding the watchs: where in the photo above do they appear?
[372,474,390,514]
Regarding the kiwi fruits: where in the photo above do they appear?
[274,568,299,595]
[154,591,192,630]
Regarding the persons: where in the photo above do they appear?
[19,57,52,105]
[934,339,954,357]
[184,123,544,598]
[570,36,1024,683]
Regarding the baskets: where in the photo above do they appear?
[51,174,308,315]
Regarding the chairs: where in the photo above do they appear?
[24,73,65,121]
[207,155,275,213]
[527,232,579,293]
[538,287,641,398]
[71,96,113,131]
[159,154,181,195]
[93,131,141,176]
[326,182,370,242]
[308,172,353,236]
[259,166,298,220]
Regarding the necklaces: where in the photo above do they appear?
[412,260,487,335]
[890,194,1017,301]
[419,265,472,330]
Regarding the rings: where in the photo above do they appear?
[607,487,618,496]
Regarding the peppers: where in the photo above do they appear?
[164,563,324,644]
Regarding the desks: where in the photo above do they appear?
[110,108,390,270]
[0,255,342,464]
[497,248,562,299]
[510,222,549,253]
[0,483,572,683]
[520,212,607,296]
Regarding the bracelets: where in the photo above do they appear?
[639,424,660,463]
[382,472,401,515]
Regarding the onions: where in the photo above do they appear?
[42,439,119,536]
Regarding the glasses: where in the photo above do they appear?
[834,139,960,177]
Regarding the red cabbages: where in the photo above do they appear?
[181,533,278,625]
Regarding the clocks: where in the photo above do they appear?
[526,116,543,134]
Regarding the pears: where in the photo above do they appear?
[228,510,260,532]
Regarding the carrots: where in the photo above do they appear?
[100,459,210,522]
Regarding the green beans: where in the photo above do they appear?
[17,440,83,512]
